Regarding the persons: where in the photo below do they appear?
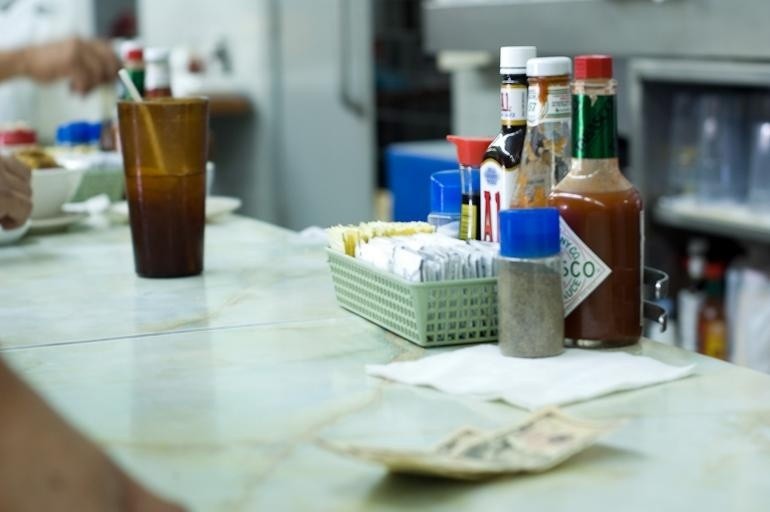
[0,146,35,233]
[0,0,123,147]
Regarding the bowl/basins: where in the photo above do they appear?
[14,148,94,222]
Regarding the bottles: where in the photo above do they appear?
[479,44,539,247]
[119,46,147,101]
[675,253,711,353]
[553,53,647,350]
[445,133,497,242]
[506,54,581,217]
[697,263,729,363]
[493,206,567,360]
[142,46,173,100]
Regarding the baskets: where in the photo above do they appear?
[326,247,498,347]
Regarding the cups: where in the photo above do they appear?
[116,97,210,280]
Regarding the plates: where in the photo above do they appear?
[0,217,32,247]
[29,212,92,236]
